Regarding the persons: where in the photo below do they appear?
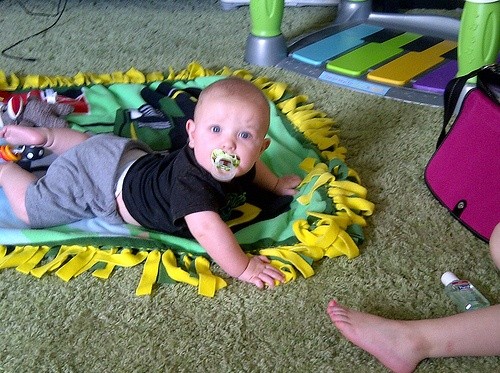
[327,223,500,373]
[0,77,302,291]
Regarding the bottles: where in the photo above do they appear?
[441,271,489,312]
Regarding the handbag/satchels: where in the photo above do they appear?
[424,64,500,243]
